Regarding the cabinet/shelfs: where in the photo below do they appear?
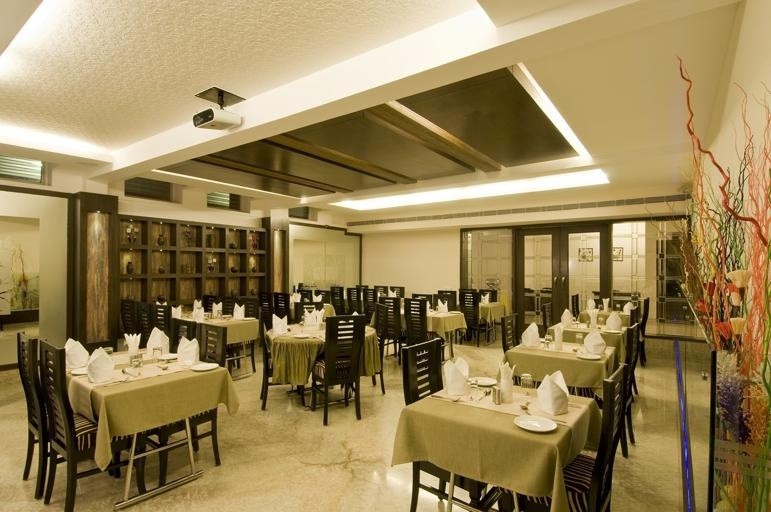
[112,215,270,319]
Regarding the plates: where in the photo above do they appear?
[468,376,498,386]
[223,315,233,318]
[192,363,220,372]
[72,367,89,375]
[159,354,180,360]
[450,310,462,314]
[577,354,601,360]
[294,333,309,338]
[243,318,256,320]
[513,415,559,432]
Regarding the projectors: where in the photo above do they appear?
[192,107,241,131]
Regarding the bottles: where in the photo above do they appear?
[126,261,134,274]
[520,373,534,396]
[158,234,165,246]
[492,386,500,405]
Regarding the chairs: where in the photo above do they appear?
[392,285,650,512]
[14,292,259,512]
[258,280,391,430]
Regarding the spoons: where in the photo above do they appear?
[122,368,138,378]
[520,401,531,410]
[478,388,492,401]
[157,365,169,370]
[430,394,462,403]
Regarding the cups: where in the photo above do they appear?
[103,346,115,360]
[470,377,479,391]
[153,347,162,366]
[543,303,621,355]
[217,309,223,323]
[204,313,209,320]
[130,354,144,374]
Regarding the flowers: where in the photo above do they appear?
[640,47,770,512]
